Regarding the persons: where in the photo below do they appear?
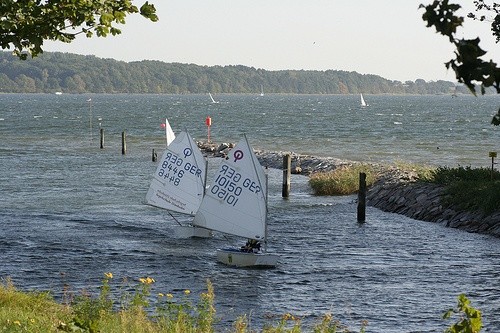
[241,238,261,253]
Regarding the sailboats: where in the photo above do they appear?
[191,133,280,268]
[207,92,219,104]
[261,84,265,96]
[143,127,217,238]
[360,94,370,107]
